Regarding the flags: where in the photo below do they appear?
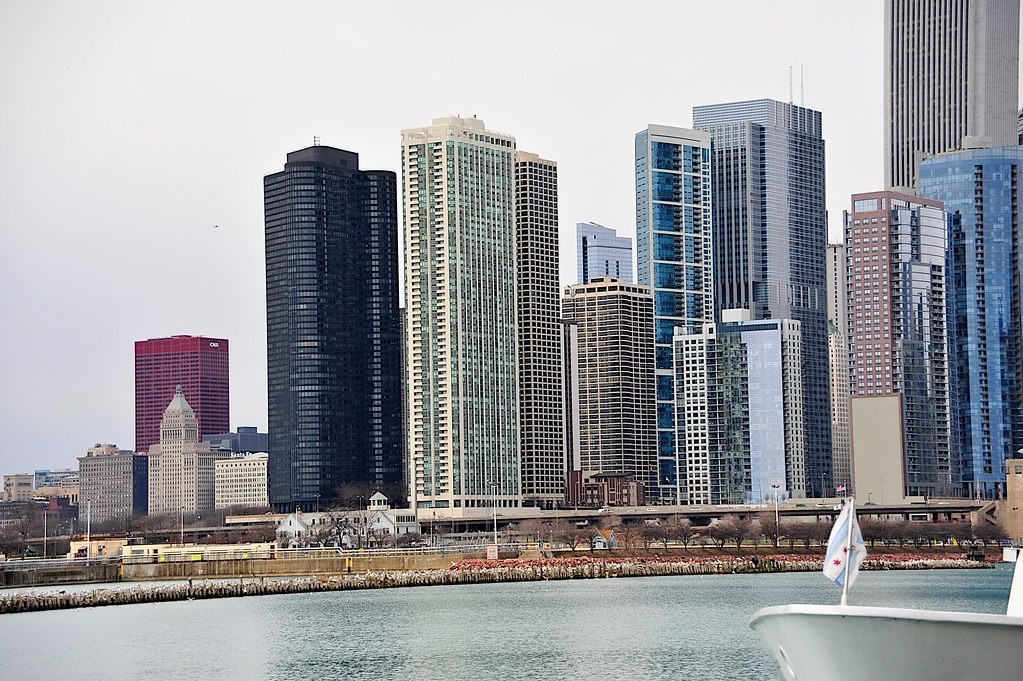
[836,483,845,492]
[822,501,868,593]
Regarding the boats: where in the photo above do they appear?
[747,492,1023,681]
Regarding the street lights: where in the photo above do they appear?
[356,495,365,529]
[314,494,321,517]
[821,472,826,498]
[771,484,781,546]
